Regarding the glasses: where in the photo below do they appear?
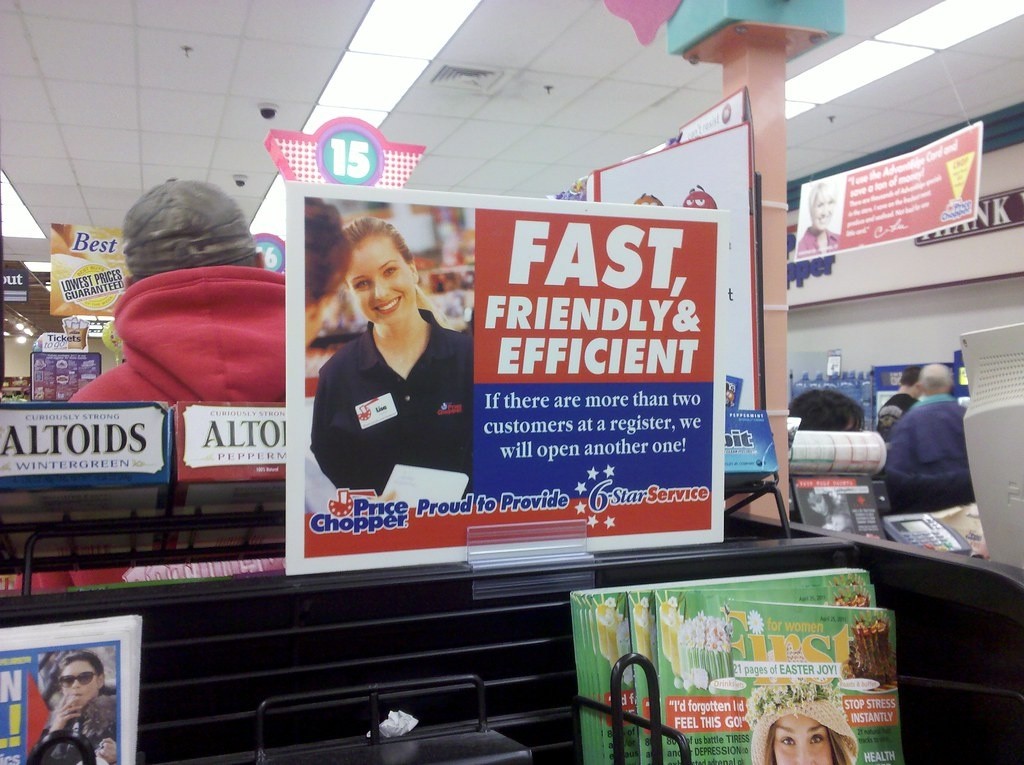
[57,671,97,688]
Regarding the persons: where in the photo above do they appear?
[70,179,287,480]
[796,181,839,259]
[800,487,854,533]
[304,196,473,503]
[790,390,865,432]
[746,684,858,765]
[876,364,975,514]
[32,651,116,764]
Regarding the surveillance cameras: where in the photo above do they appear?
[259,103,278,119]
[233,175,247,186]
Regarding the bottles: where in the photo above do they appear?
[792,371,873,423]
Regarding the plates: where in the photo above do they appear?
[840,661,898,694]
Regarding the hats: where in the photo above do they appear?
[121,177,257,279]
[745,684,858,765]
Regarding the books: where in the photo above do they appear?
[792,474,886,539]
[570,568,904,765]
[0,615,142,765]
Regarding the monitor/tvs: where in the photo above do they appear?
[958,322,1024,570]
